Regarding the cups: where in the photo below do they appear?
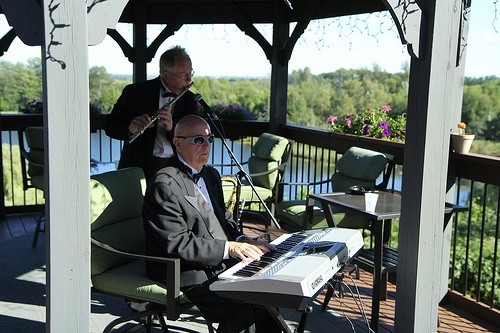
[364,193,379,212]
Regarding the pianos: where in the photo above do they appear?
[207,227,363,312]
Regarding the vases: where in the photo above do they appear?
[450,134,475,155]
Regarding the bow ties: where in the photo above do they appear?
[193,166,209,184]
[162,92,177,98]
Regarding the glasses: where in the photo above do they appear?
[159,69,194,80]
[175,134,214,145]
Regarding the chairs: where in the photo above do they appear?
[275,144,398,282]
[222,132,295,235]
[16,124,45,248]
[90,167,242,332]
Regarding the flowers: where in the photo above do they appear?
[456,122,466,135]
[210,104,260,122]
[327,105,407,142]
[89,99,113,114]
[15,92,43,114]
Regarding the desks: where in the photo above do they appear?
[309,189,469,333]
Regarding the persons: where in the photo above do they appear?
[143,115,279,333]
[105,45,204,183]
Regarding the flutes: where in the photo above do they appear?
[127,81,196,146]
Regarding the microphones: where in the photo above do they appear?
[194,93,218,120]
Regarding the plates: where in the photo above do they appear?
[350,185,373,196]
